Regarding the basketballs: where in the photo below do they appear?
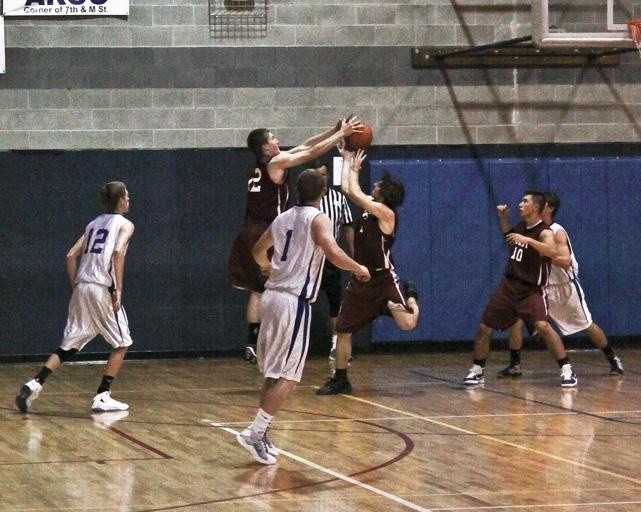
[344,121,373,152]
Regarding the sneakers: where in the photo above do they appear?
[404,280,416,294]
[237,427,280,465]
[498,366,522,379]
[91,391,129,412]
[610,355,624,375]
[463,364,484,384]
[243,345,258,363]
[15,379,42,412]
[560,364,578,388]
[317,378,351,395]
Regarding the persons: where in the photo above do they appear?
[228,112,364,364]
[24,410,134,512]
[315,142,420,396]
[235,465,301,512]
[462,376,626,512]
[464,190,577,387]
[497,192,624,379]
[235,168,372,465]
[15,181,135,411]
[309,159,354,362]
